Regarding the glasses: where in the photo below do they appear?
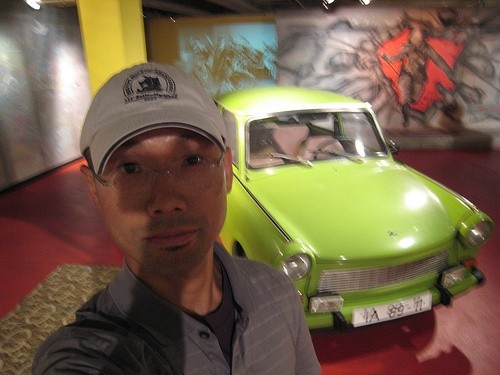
[87,153,223,194]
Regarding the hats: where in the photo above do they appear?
[80,64,227,176]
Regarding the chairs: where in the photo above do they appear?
[249,124,342,168]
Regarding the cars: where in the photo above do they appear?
[214,85,496,330]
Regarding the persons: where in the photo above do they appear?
[32,62,322,375]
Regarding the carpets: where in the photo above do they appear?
[0,262,121,375]
